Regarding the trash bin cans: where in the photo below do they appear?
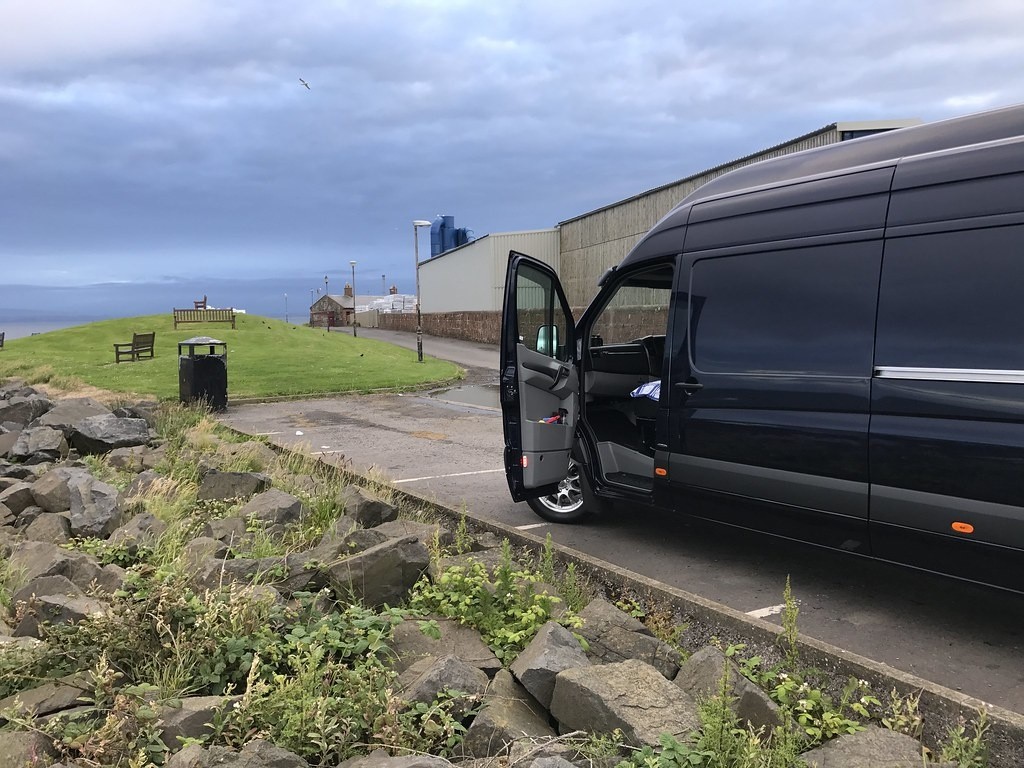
[177,336,228,414]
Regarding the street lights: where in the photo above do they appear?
[350,261,357,337]
[412,220,432,363]
[284,293,288,323]
[323,275,330,333]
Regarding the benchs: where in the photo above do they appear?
[173,307,236,331]
[113,332,155,363]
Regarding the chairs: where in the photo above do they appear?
[194,295,207,310]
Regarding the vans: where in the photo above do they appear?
[497,99,1023,598]
